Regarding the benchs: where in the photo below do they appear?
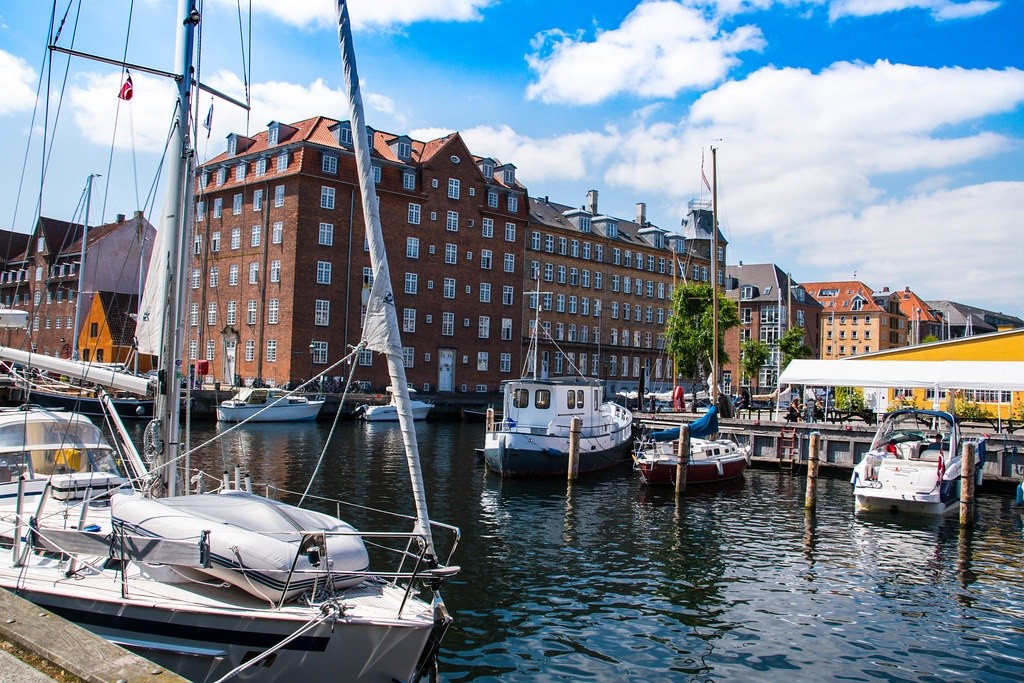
[793,410,877,428]
[923,418,1024,434]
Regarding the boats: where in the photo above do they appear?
[0,0,462,683]
[212,186,325,422]
[8,173,199,417]
[631,138,753,485]
[475,269,633,476]
[361,386,436,421]
[851,409,987,515]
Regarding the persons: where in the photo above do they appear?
[886,438,901,459]
[814,396,825,422]
[789,399,808,423]
[919,433,944,458]
[731,386,749,419]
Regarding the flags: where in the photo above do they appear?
[937,448,946,479]
[202,103,213,140]
[117,76,133,100]
